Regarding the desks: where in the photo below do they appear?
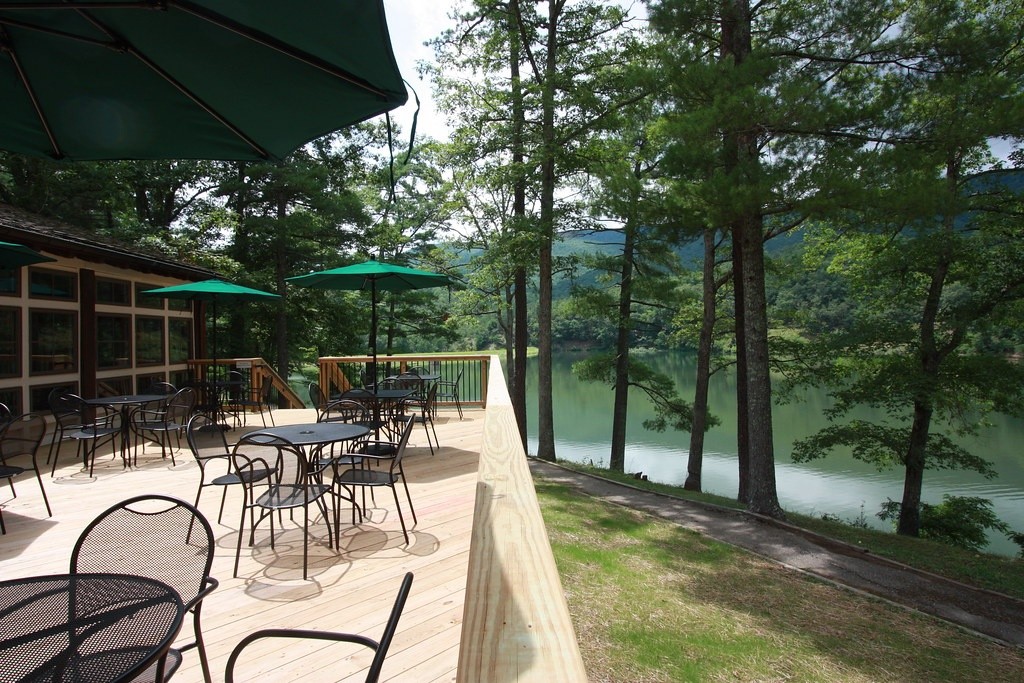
[0,573,185,683]
[385,375,441,383]
[189,381,241,438]
[241,423,370,549]
[330,389,417,399]
[80,395,169,467]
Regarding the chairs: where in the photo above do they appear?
[0,370,465,683]
